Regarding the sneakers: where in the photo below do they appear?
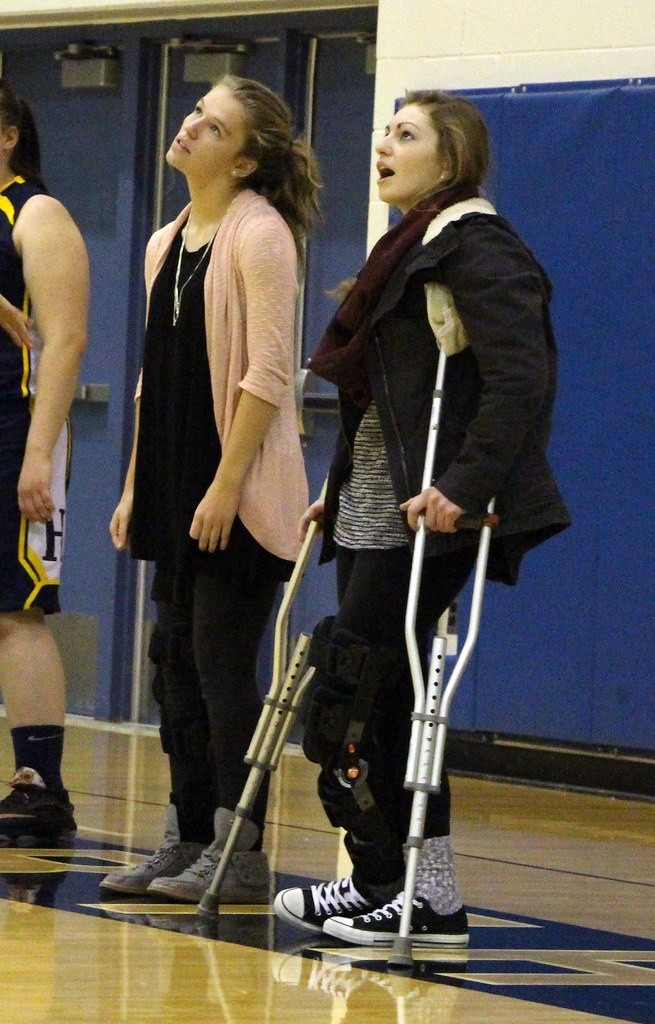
[274,878,380,936]
[145,807,269,903]
[0,766,78,844]
[321,883,470,949]
[98,801,212,893]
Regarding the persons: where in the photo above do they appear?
[98,75,324,906]
[1,88,90,841]
[298,93,577,949]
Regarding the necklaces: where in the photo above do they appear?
[170,206,226,326]
